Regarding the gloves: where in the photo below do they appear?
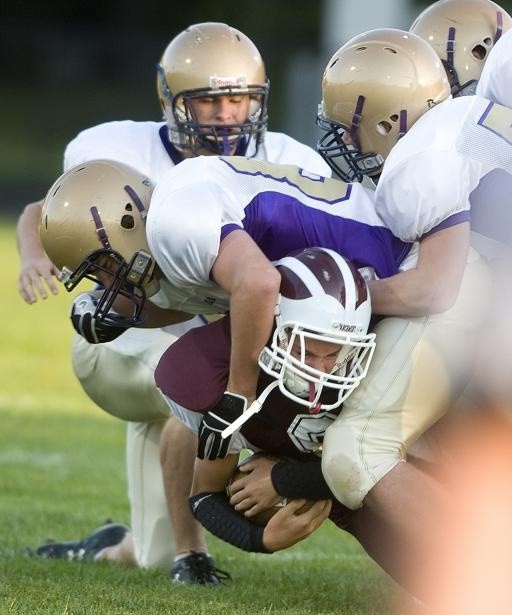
[195,392,248,461]
[69,293,126,346]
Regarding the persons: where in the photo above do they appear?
[42,2,511,614]
[15,23,333,588]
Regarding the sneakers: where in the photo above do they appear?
[169,550,233,586]
[35,521,130,562]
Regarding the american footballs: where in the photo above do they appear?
[226,451,346,525]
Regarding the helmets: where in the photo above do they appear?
[255,245,377,414]
[38,159,157,329]
[407,1,512,99]
[314,28,451,189]
[154,22,271,160]
